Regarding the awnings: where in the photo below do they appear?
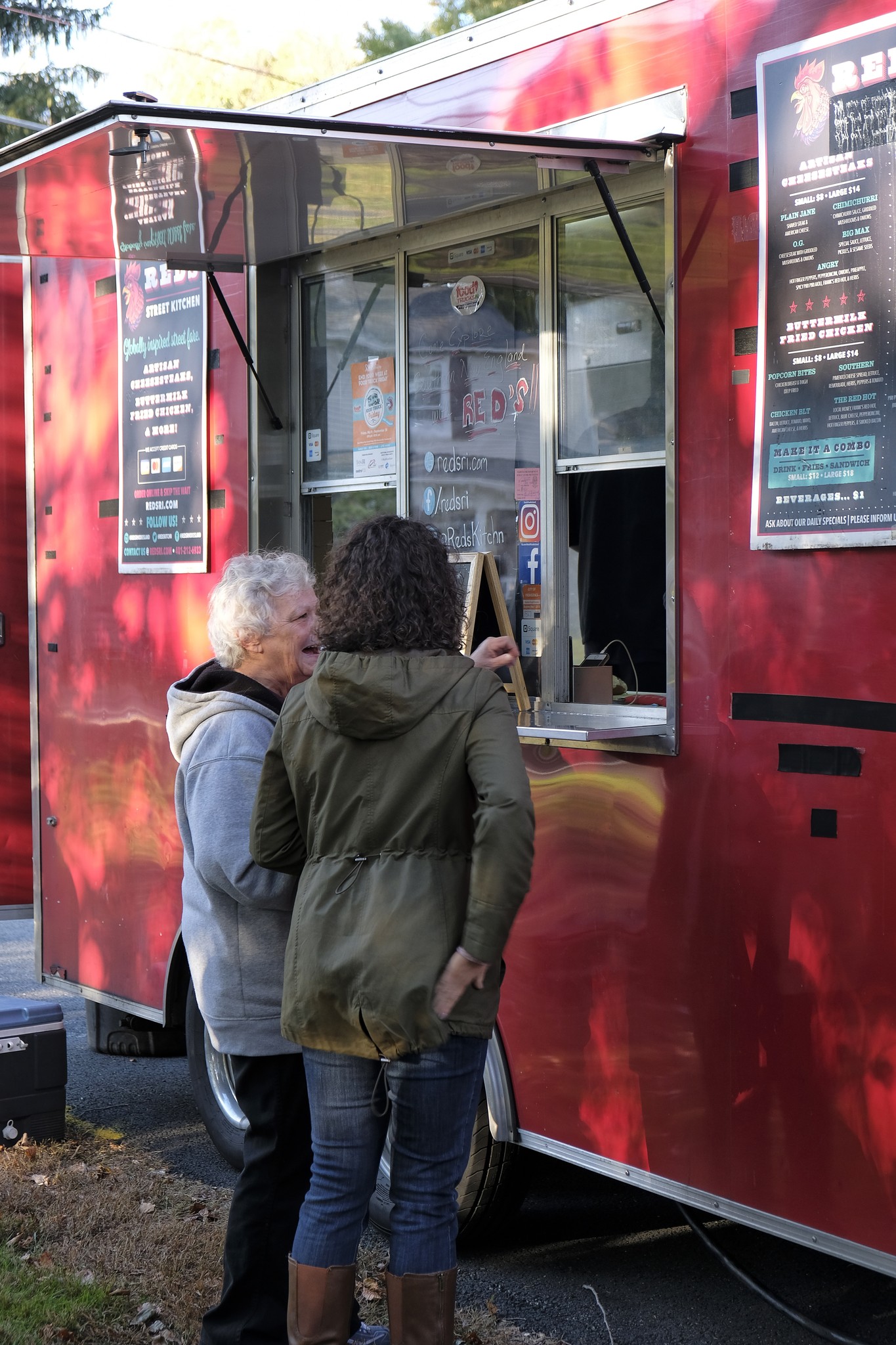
[0,100,668,431]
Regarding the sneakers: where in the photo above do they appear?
[347,1323,389,1345]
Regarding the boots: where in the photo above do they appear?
[383,1261,455,1345]
[287,1252,356,1345]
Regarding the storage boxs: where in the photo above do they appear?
[83,937,190,1059]
[571,664,614,705]
[0,995,70,1149]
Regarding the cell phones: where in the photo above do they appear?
[579,653,609,667]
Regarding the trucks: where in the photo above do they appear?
[0,1,896,1281]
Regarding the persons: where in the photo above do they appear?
[170,554,523,1345]
[249,518,536,1345]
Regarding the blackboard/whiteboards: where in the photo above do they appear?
[444,550,525,694]
